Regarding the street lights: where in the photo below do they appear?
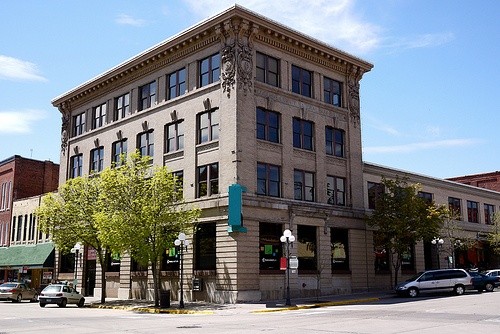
[431,236,444,270]
[71,243,84,291]
[174,233,189,308]
[280,229,295,306]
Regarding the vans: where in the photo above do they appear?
[395,269,473,298]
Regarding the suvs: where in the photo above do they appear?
[483,269,500,285]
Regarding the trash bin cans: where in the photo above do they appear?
[159,289,171,308]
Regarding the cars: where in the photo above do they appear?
[0,282,38,303]
[39,284,85,308]
[465,272,500,291]
